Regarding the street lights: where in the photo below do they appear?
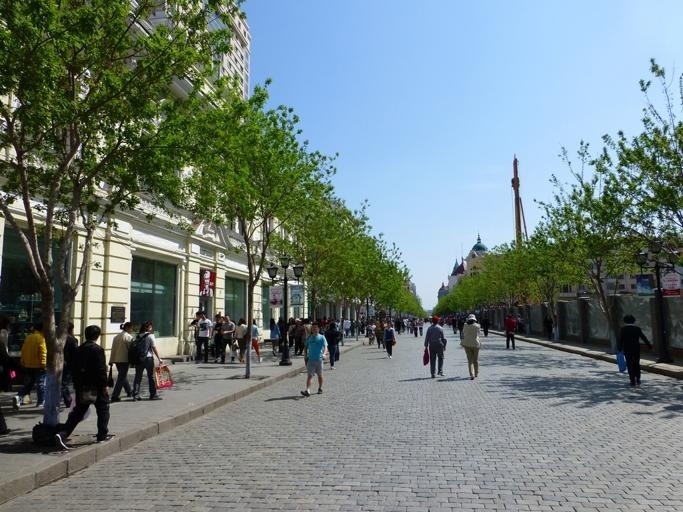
[266,252,304,366]
[361,295,373,326]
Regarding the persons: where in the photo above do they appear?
[483,316,491,337]
[545,315,553,340]
[301,322,328,396]
[439,315,465,333]
[617,314,653,386]
[0,315,162,447]
[190,312,312,363]
[425,316,447,378]
[504,314,517,350]
[462,314,481,380]
[321,316,424,369]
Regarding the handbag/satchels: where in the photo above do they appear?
[617,351,626,371]
[156,362,173,388]
[107,377,114,388]
[423,349,429,365]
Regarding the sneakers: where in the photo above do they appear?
[317,389,322,394]
[55,433,69,449]
[99,434,112,442]
[630,381,635,385]
[111,390,162,401]
[37,402,44,407]
[300,390,310,396]
[195,358,226,364]
[637,379,641,384]
[12,395,21,411]
[470,375,475,379]
[438,373,446,376]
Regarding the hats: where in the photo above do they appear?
[433,316,441,321]
[467,314,477,321]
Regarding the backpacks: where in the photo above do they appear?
[128,333,152,365]
